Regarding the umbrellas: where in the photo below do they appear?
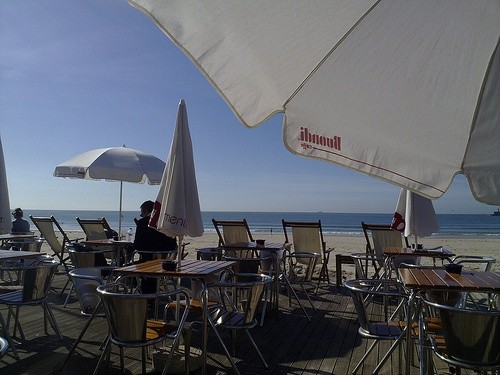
[389,187,440,262]
[125,0,500,207]
[148,98,204,269]
[53,143,167,241]
[0,137,13,236]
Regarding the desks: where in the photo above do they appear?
[0,235,37,286]
[0,249,46,262]
[399,268,500,375]
[112,258,236,375]
[81,238,135,266]
[381,246,456,311]
[223,242,292,312]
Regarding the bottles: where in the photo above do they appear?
[128,227,134,242]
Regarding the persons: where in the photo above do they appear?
[11,207,31,264]
[133,200,179,302]
[127,227,134,242]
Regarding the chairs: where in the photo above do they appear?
[0,215,500,375]
